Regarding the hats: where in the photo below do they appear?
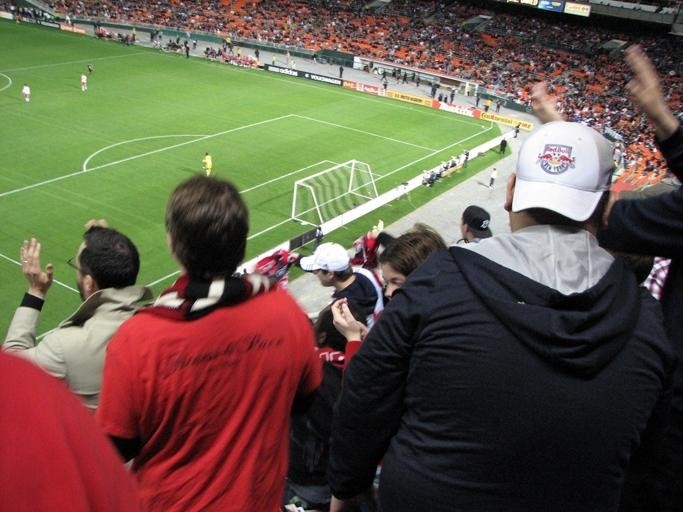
[463,205,493,238]
[511,121,617,222]
[300,242,350,272]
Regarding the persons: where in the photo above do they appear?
[1,0,682,511]
